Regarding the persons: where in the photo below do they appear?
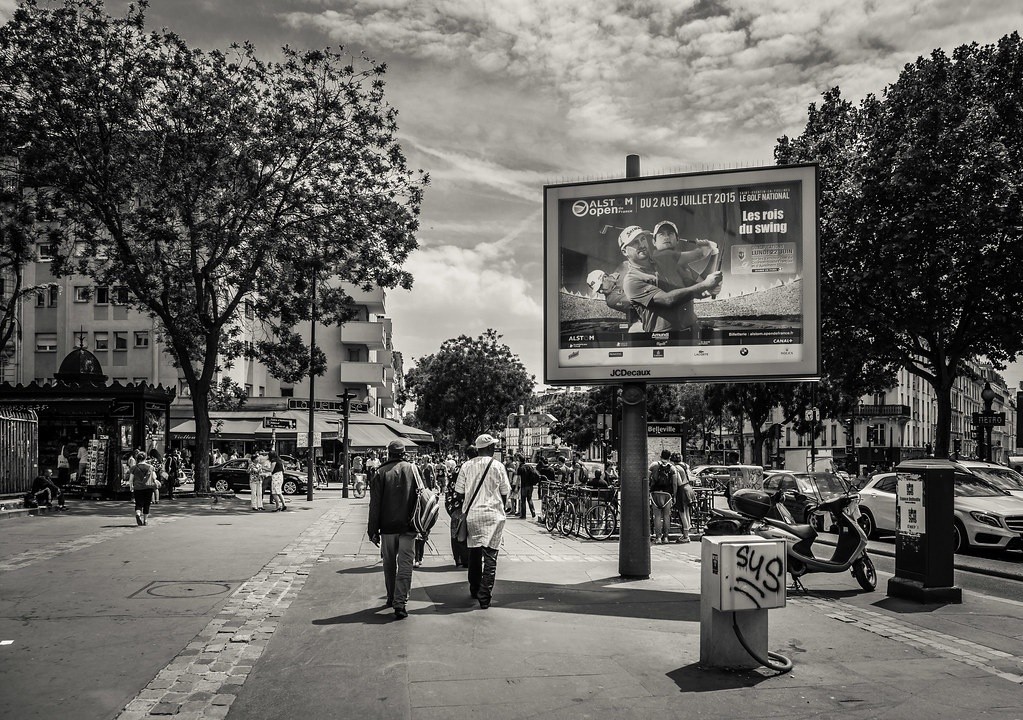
[871,464,884,475]
[586,220,724,330]
[587,462,618,497]
[669,451,692,542]
[128,451,155,525]
[31,467,69,510]
[353,453,364,473]
[127,448,183,504]
[248,451,287,512]
[76,439,89,469]
[455,434,511,608]
[649,450,677,543]
[502,453,536,519]
[419,454,457,493]
[214,449,238,465]
[444,445,479,568]
[729,451,742,471]
[537,451,588,499]
[367,440,426,617]
[366,451,381,470]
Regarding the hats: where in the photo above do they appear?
[587,270,605,293]
[476,433,499,448]
[389,439,405,454]
[652,220,679,246]
[617,225,651,250]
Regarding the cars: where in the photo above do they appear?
[956,460,1023,502]
[208,458,318,496]
[857,471,1023,555]
[760,471,860,532]
[692,465,731,489]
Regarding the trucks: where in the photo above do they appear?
[524,445,574,484]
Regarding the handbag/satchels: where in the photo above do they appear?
[528,464,540,486]
[454,515,467,542]
[145,468,160,489]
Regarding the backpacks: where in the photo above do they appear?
[657,461,673,486]
[576,462,588,483]
[412,464,439,539]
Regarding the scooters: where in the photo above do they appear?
[729,478,878,595]
[699,487,807,541]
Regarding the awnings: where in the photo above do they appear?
[168,410,434,453]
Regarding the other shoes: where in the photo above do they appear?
[251,499,287,511]
[136,514,143,526]
[652,538,662,544]
[520,516,526,520]
[675,535,690,543]
[481,602,490,608]
[532,511,535,517]
[395,606,408,617]
[386,599,394,607]
[456,559,463,566]
[150,501,159,505]
[144,521,148,526]
[663,536,668,544]
[415,560,422,567]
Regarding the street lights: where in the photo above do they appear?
[979,381,994,462]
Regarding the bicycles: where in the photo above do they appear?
[541,480,591,537]
[584,485,672,541]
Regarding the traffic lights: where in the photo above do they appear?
[867,425,875,442]
[347,393,357,400]
[335,388,347,417]
[954,438,962,452]
